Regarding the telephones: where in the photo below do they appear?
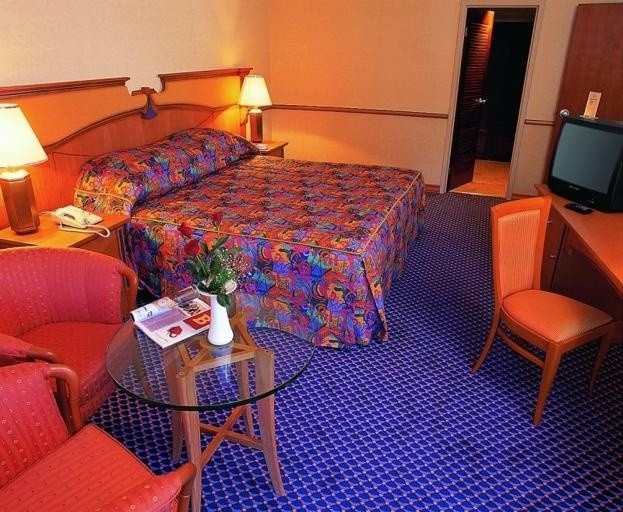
[50,205,103,229]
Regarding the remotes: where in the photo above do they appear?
[565,203,593,215]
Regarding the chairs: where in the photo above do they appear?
[1,363,195,512]
[0,246,157,423]
[473,197,616,426]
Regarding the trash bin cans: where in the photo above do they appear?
[475,128,494,160]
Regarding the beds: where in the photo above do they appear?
[1,68,425,349]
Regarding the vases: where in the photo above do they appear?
[196,278,238,347]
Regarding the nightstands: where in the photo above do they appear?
[0,206,130,290]
[248,140,289,158]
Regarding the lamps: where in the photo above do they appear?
[0,104,49,234]
[238,75,271,143]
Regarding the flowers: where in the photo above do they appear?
[193,234,248,307]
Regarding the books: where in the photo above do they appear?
[130,287,213,349]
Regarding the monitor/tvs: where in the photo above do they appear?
[547,116,623,213]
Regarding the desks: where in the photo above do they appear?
[533,180,622,340]
[102,288,317,509]
